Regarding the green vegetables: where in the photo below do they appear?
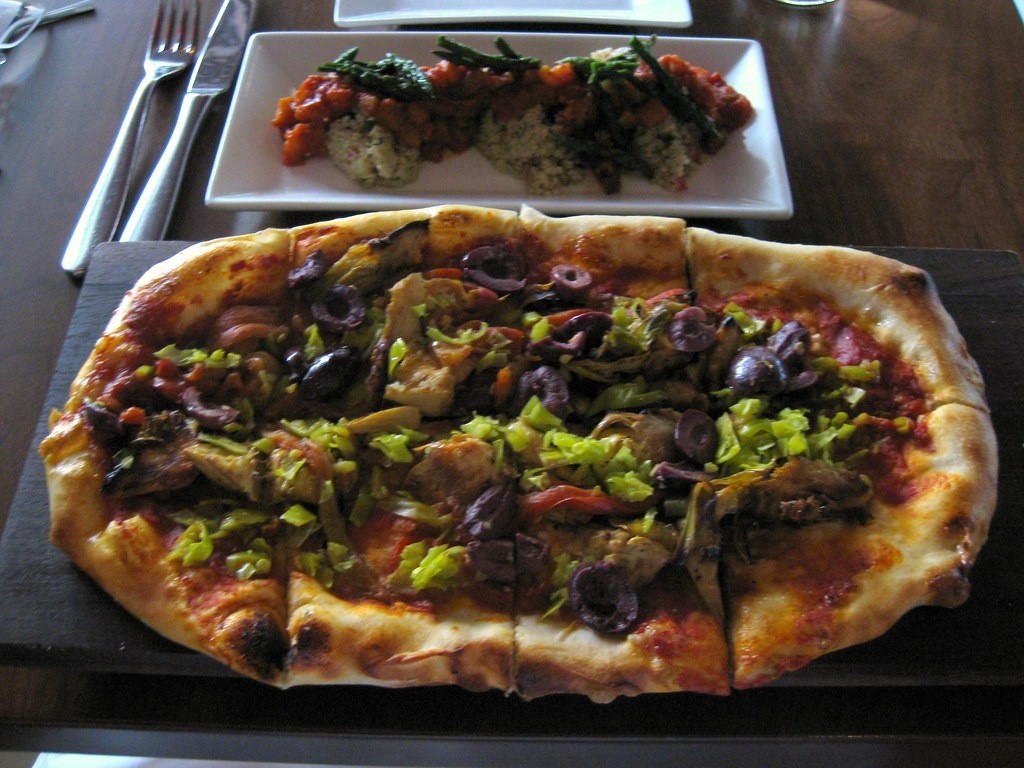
[314,35,725,198]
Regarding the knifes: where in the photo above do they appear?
[118,0,259,242]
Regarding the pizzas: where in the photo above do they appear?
[38,206,1000,704]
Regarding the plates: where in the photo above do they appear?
[205,31,794,219]
[332,0,693,28]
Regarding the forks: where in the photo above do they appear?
[62,0,202,276]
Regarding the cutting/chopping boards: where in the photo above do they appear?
[1,239,1024,704]
